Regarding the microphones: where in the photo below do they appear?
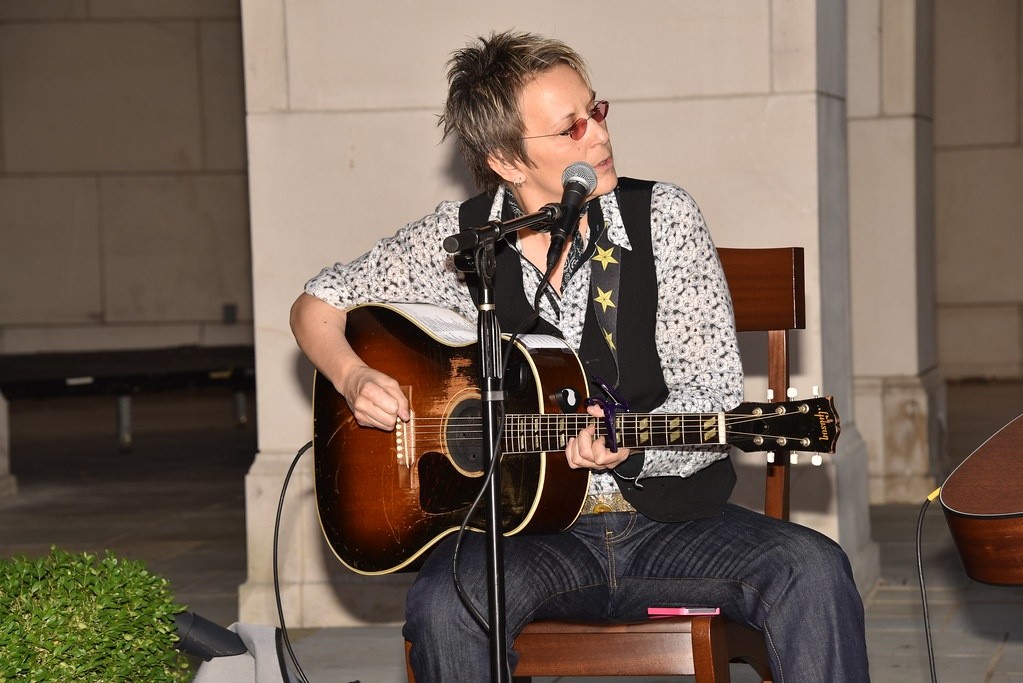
[545,161,596,265]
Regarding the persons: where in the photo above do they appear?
[290,29,873,682]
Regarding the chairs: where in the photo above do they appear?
[403,246,806,678]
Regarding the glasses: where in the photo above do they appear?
[519,100,609,140]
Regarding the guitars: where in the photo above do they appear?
[939,412,1022,586]
[313,303,840,576]
